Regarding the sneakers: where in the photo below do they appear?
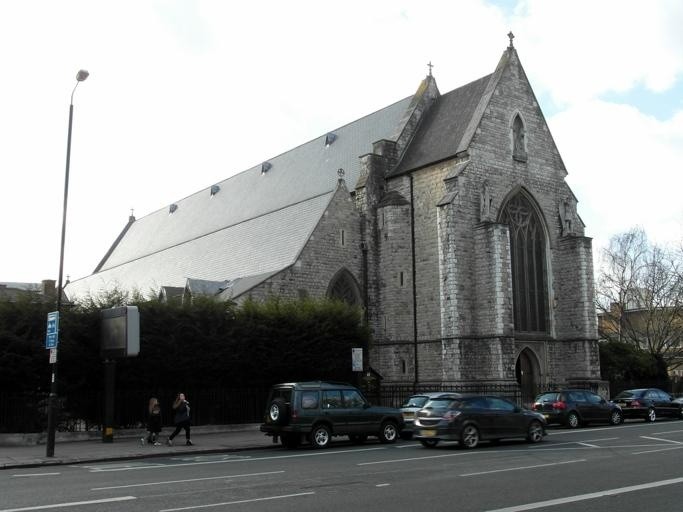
[167,437,173,445]
[141,439,145,445]
[186,441,193,445]
[154,442,162,446]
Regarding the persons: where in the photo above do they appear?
[141,397,163,445]
[164,393,194,447]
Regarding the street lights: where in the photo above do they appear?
[48,70,90,461]
[404,169,422,382]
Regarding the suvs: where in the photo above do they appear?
[260,382,405,449]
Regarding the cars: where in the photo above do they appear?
[531,389,624,429]
[609,388,683,422]
[398,392,548,448]
